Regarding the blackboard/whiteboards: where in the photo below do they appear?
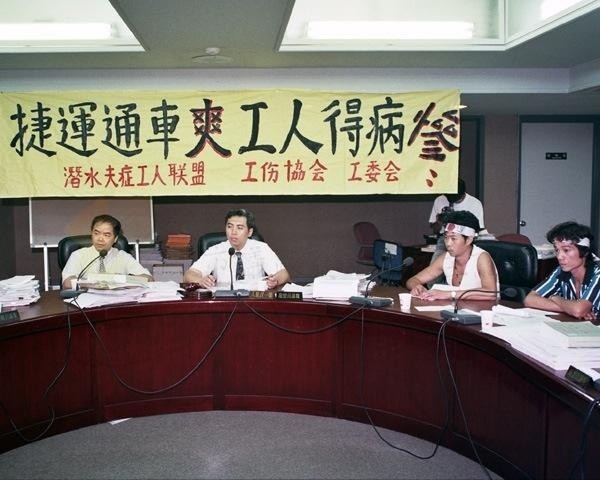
[29,197,155,249]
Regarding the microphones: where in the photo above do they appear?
[440,288,517,325]
[349,257,414,308]
[60,249,107,297]
[215,248,249,297]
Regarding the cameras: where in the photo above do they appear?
[437,206,454,224]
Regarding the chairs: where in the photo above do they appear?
[373,238,405,283]
[473,243,537,302]
[57,233,131,272]
[197,229,264,261]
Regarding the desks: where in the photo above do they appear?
[0,282,600,479]
[399,244,560,288]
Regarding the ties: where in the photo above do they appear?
[99,256,105,272]
[235,252,244,280]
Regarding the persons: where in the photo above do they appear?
[405,209,501,303]
[182,208,291,288]
[521,220,600,321]
[425,178,485,288]
[61,214,153,290]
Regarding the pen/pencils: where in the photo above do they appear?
[263,274,276,279]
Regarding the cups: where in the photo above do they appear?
[71,279,80,291]
[398,292,411,309]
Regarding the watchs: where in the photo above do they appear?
[451,291,457,300]
